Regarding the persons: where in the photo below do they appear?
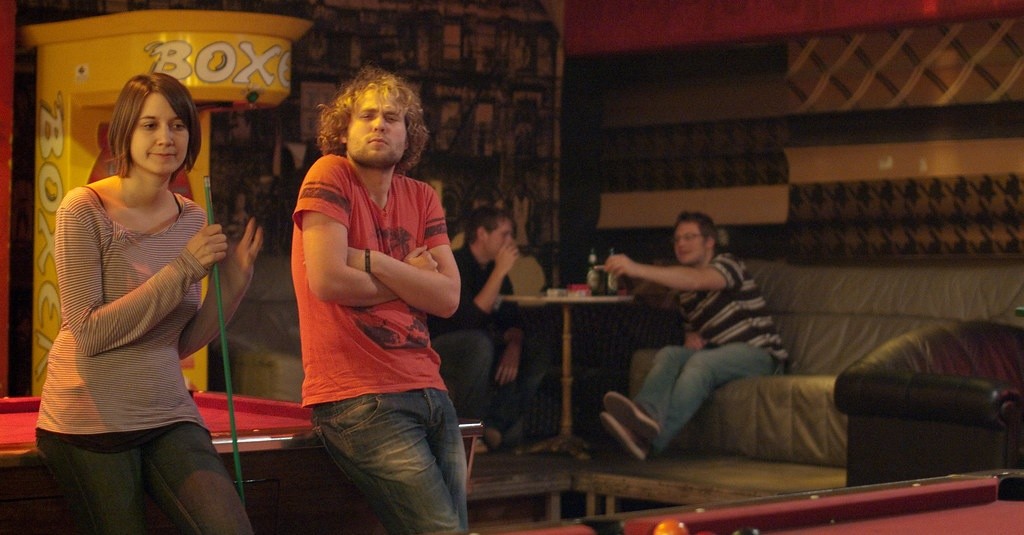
[599,212,796,461]
[429,207,550,446]
[291,69,469,535]
[36,73,263,535]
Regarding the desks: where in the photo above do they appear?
[424,469,1024,535]
[497,294,633,460]
[0,388,484,535]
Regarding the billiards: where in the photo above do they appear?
[653,518,689,535]
[733,527,760,535]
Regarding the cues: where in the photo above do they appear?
[203,172,246,513]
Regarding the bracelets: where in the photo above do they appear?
[365,250,370,273]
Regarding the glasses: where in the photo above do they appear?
[669,233,708,247]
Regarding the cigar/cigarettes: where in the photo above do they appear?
[594,265,605,269]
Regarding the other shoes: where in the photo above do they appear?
[484,427,502,447]
[466,438,487,453]
[599,412,650,462]
[603,391,660,438]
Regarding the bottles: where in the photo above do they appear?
[605,247,619,295]
[586,248,599,295]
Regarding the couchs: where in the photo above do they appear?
[833,317,1024,488]
[629,260,1024,468]
